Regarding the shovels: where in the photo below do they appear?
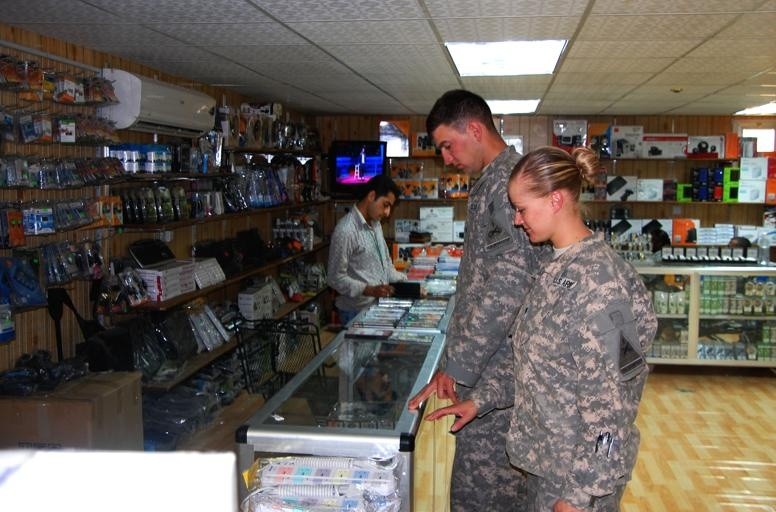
[47,287,107,362]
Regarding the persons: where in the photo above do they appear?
[328,332,399,418]
[726,235,752,262]
[325,175,429,326]
[419,143,657,512]
[406,86,551,511]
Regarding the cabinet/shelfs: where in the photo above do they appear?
[107,147,468,512]
[584,148,776,368]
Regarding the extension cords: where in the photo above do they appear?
[253,457,397,512]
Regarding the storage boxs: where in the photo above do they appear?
[1,371,144,453]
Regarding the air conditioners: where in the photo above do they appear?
[98,67,216,141]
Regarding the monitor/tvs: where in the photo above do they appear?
[327,140,387,198]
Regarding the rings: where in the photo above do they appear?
[386,293,390,296]
